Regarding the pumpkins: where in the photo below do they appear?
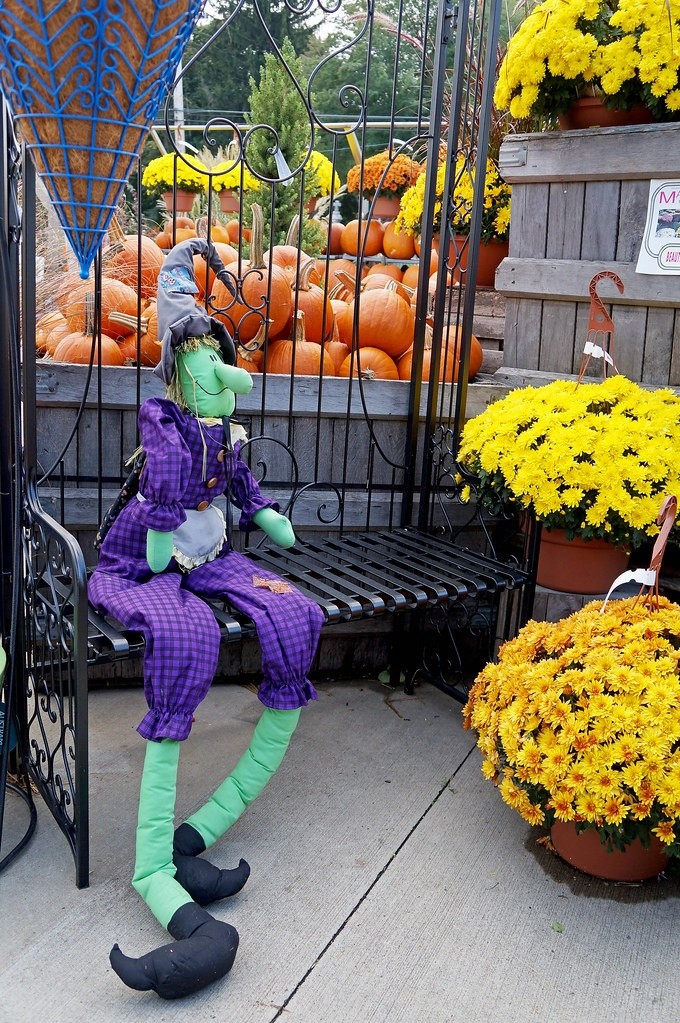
[35,202,484,383]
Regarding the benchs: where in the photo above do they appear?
[20,473,540,886]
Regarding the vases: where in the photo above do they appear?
[573,96,644,127]
[432,229,508,287]
[303,196,319,213]
[219,189,242,215]
[370,193,404,218]
[160,189,195,214]
[550,803,664,880]
[518,511,631,594]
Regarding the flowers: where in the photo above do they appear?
[461,381,680,544]
[346,152,418,190]
[396,156,506,235]
[142,151,209,194]
[495,0,680,130]
[299,150,340,196]
[211,160,260,191]
[462,596,680,846]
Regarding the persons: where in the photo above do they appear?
[83,237,326,1000]
